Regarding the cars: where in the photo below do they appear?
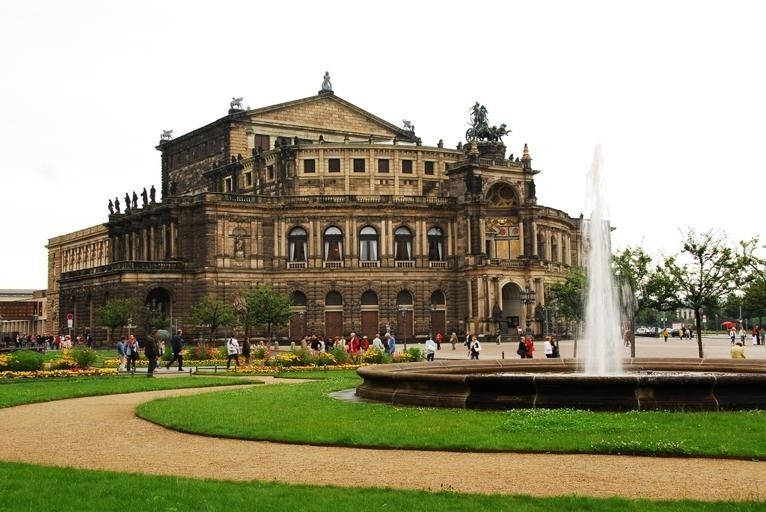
[632,324,693,337]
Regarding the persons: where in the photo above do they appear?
[730,340,747,358]
[241,337,250,364]
[225,334,241,369]
[425,336,436,360]
[165,329,184,372]
[465,332,471,357]
[663,328,670,342]
[678,326,693,340]
[470,336,482,360]
[299,332,396,358]
[728,325,766,346]
[495,333,501,355]
[123,335,139,374]
[515,332,560,359]
[435,332,443,350]
[117,336,126,369]
[12,334,91,349]
[450,332,458,350]
[143,330,160,374]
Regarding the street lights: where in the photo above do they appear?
[126,318,132,340]
[520,285,536,341]
[402,310,407,352]
[287,311,303,339]
